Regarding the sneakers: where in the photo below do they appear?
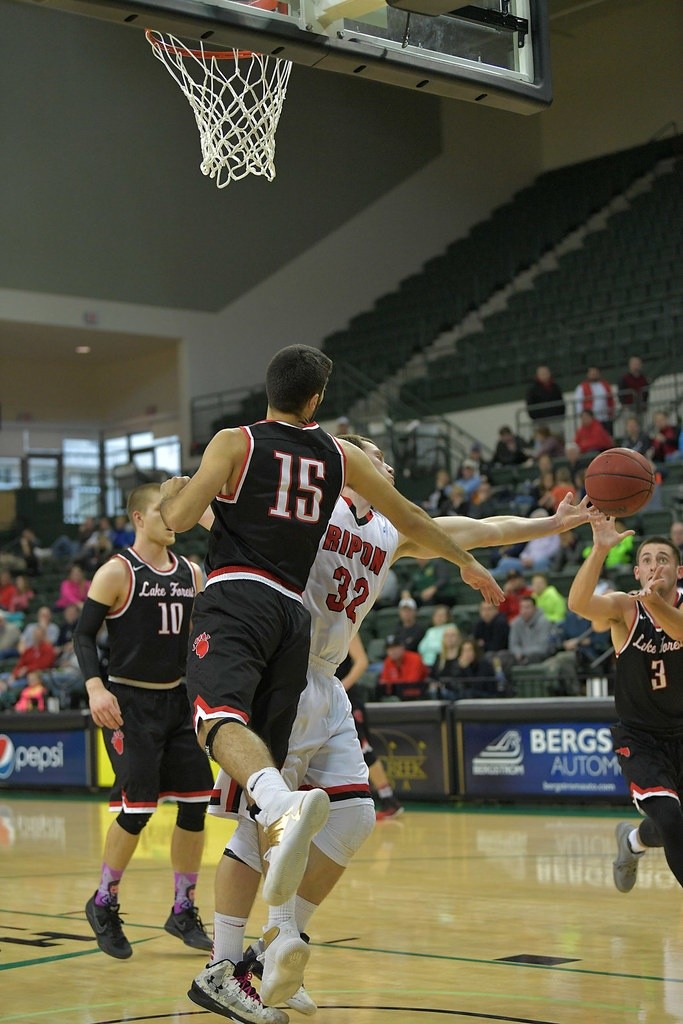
[613,823,646,892]
[187,958,290,1024]
[249,787,329,907]
[85,888,132,958]
[259,917,310,1006]
[245,943,317,1016]
[164,905,213,951]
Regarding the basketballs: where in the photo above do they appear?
[581,444,656,519]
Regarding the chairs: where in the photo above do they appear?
[0,442,683,714]
[208,134,683,440]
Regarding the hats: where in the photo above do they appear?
[399,598,418,610]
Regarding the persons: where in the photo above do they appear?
[72,482,215,960]
[332,629,406,823]
[185,429,606,1024]
[377,352,683,699]
[158,344,510,1008]
[0,516,134,711]
[566,513,683,894]
